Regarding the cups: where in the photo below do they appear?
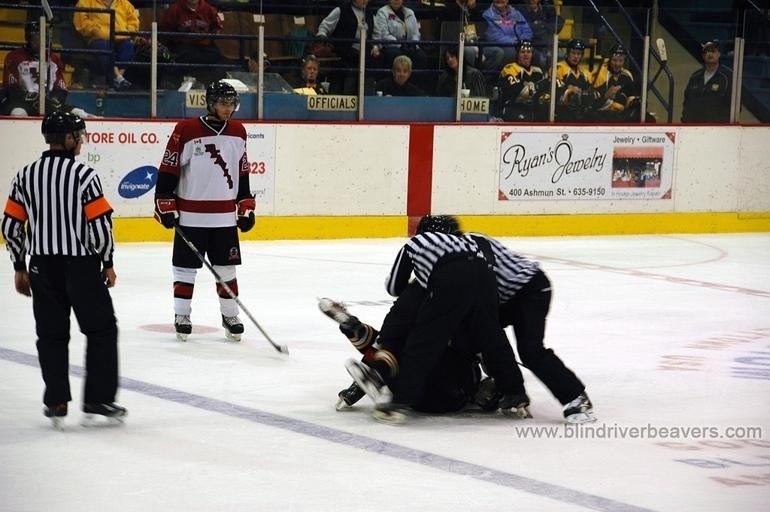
[376,89,383,97]
[460,89,470,98]
[321,82,330,92]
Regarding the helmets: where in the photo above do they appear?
[416,213,459,234]
[610,44,627,64]
[567,39,585,56]
[41,109,86,144]
[205,79,239,114]
[517,38,535,55]
[25,20,49,42]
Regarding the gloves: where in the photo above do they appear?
[153,195,180,228]
[236,197,256,233]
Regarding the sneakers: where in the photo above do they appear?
[82,402,126,416]
[121,79,132,88]
[177,80,192,93]
[175,314,192,334]
[319,299,592,423]
[42,403,67,417]
[222,315,244,334]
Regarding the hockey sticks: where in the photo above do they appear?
[41,1,55,113]
[642,38,667,88]
[172,223,289,354]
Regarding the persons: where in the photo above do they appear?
[681,38,743,123]
[155,81,257,336]
[73,1,141,91]
[160,0,227,85]
[316,213,595,425]
[2,112,131,417]
[3,20,97,118]
